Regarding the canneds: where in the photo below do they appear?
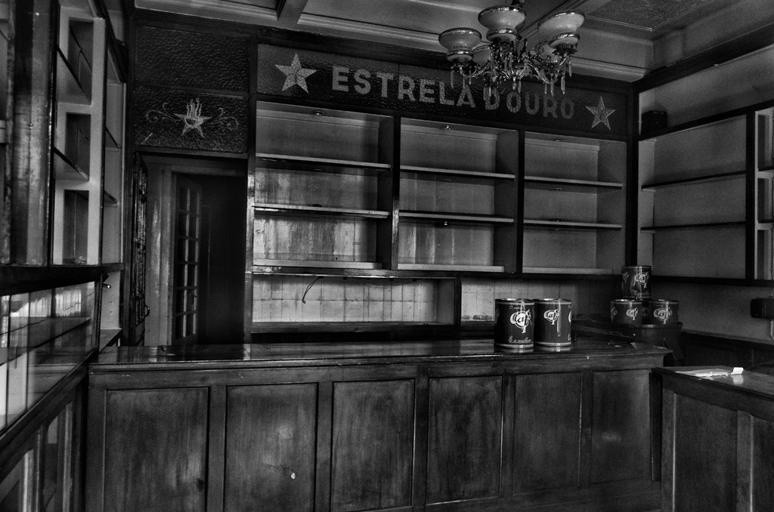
[610,298,643,325]
[622,265,651,301]
[648,298,680,324]
[493,297,536,353]
[534,297,573,352]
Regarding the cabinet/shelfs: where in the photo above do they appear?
[82,352,665,512]
[250,90,631,280]
[633,24,774,288]
[652,372,771,511]
[0,2,153,512]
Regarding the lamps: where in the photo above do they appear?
[436,1,586,96]
